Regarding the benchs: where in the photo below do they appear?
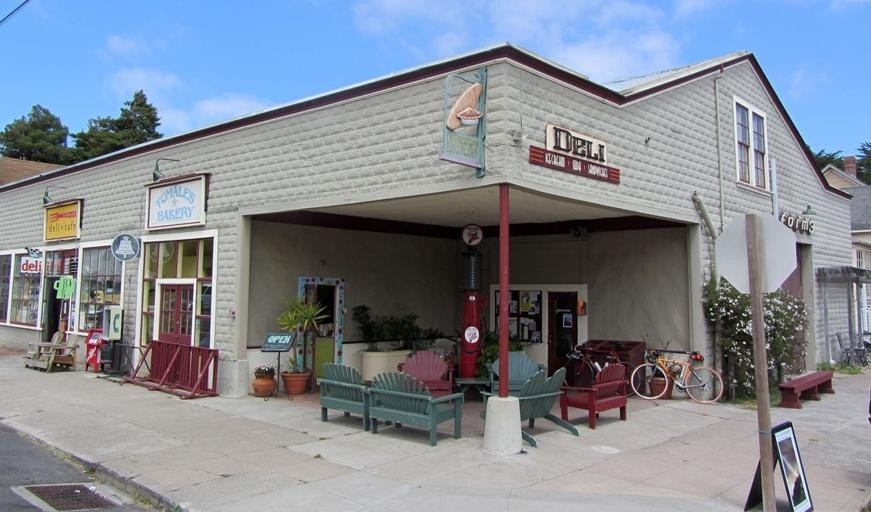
[777,370,836,408]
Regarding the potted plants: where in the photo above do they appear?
[272,296,331,396]
[475,340,523,396]
[252,364,277,397]
[646,351,674,400]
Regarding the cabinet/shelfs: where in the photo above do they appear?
[576,339,645,397]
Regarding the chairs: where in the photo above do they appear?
[400,350,455,405]
[561,362,627,429]
[316,362,391,431]
[367,371,463,447]
[24,332,65,370]
[480,366,579,448]
[38,333,79,373]
[485,350,539,393]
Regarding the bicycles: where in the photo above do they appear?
[558,340,724,406]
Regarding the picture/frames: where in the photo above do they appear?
[744,431,778,512]
[771,422,814,512]
